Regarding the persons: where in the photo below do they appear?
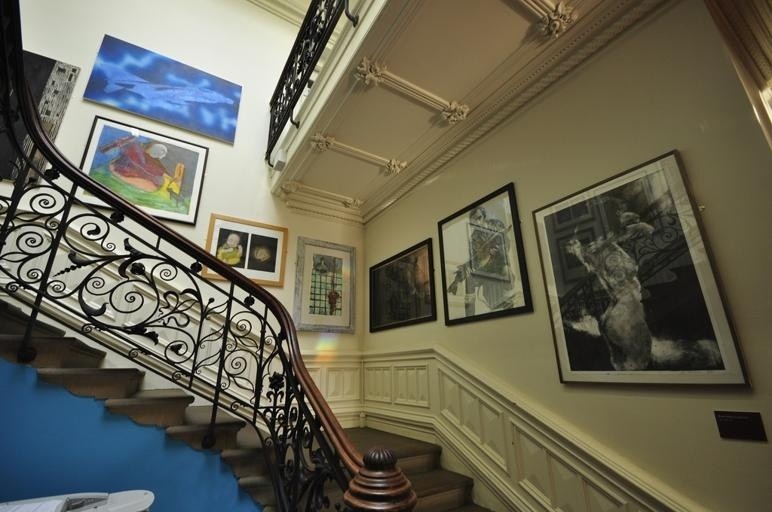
[314,257,328,272]
[216,233,242,266]
[454,206,515,317]
[329,289,339,316]
[108,140,184,202]
[567,212,656,372]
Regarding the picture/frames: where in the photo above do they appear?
[531,148,754,398]
[435,181,533,327]
[69,115,210,229]
[369,237,437,332]
[291,234,357,335]
[200,213,289,289]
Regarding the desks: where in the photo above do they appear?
[63,488,155,512]
